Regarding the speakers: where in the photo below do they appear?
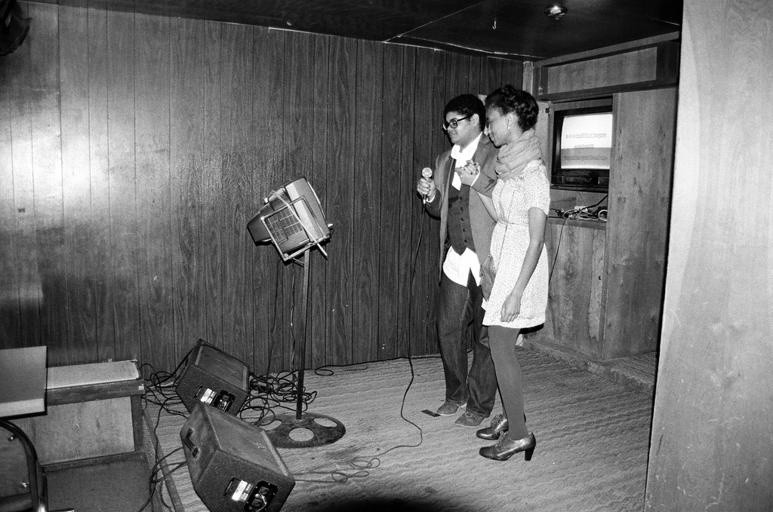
[176,339,249,415]
[181,402,294,511]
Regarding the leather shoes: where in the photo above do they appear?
[437,399,467,416]
[455,411,484,428]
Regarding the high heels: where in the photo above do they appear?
[476,413,536,461]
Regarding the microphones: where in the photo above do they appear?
[420,167,433,181]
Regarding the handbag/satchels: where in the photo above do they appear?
[480,253,497,301]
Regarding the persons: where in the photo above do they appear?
[462,86,552,460]
[415,92,498,425]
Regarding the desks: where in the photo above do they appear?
[0,345,47,511]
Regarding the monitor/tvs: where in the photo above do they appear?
[554,110,612,184]
[249,179,331,253]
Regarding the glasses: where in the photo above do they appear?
[442,115,469,131]
